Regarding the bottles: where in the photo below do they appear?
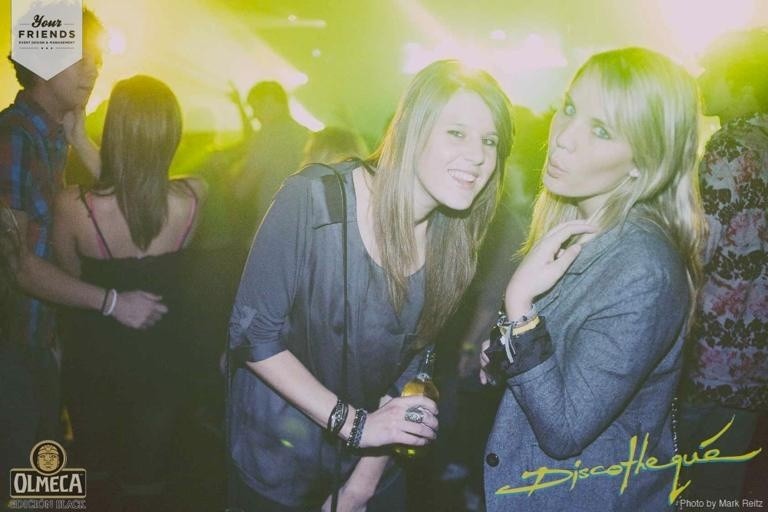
[394,349,440,460]
[484,300,509,387]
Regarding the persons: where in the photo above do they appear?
[0,2,766,509]
